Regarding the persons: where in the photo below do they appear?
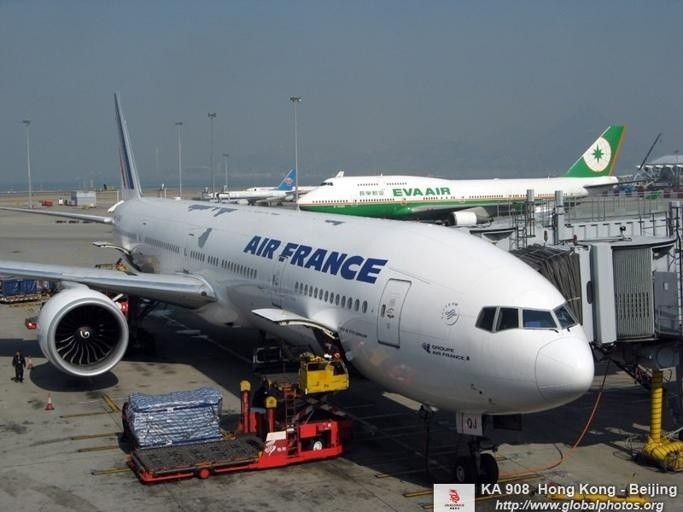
[252,380,269,408]
[11,350,26,383]
[323,339,340,362]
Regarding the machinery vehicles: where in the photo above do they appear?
[126,335,355,487]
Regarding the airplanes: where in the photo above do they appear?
[206,169,346,206]
[295,123,626,219]
[0,88,595,492]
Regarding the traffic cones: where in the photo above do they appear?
[45,393,57,412]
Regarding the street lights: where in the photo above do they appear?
[206,112,216,167]
[288,94,303,209]
[20,119,32,210]
[222,153,229,189]
[174,120,183,198]
